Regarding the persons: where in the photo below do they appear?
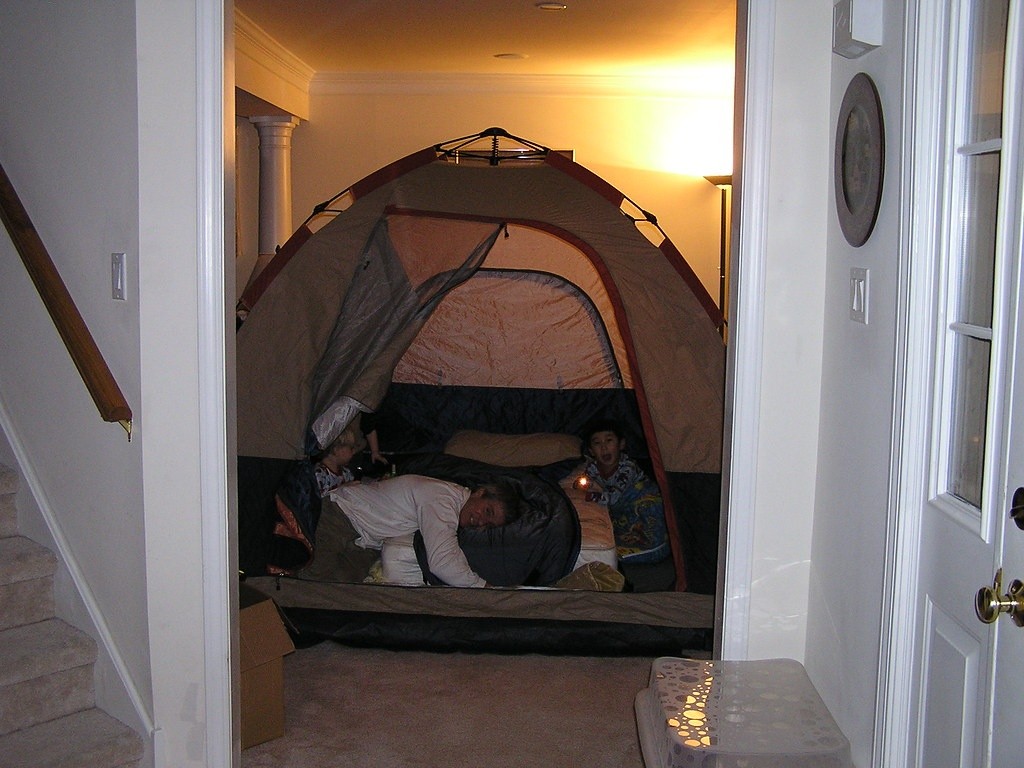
[305,403,433,497]
[573,419,670,562]
[272,472,523,591]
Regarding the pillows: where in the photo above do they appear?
[442,428,586,468]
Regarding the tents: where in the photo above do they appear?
[237,126,734,658]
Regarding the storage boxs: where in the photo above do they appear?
[237,581,300,749]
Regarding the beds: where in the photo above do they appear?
[383,455,617,587]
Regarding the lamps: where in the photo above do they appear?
[702,175,732,339]
